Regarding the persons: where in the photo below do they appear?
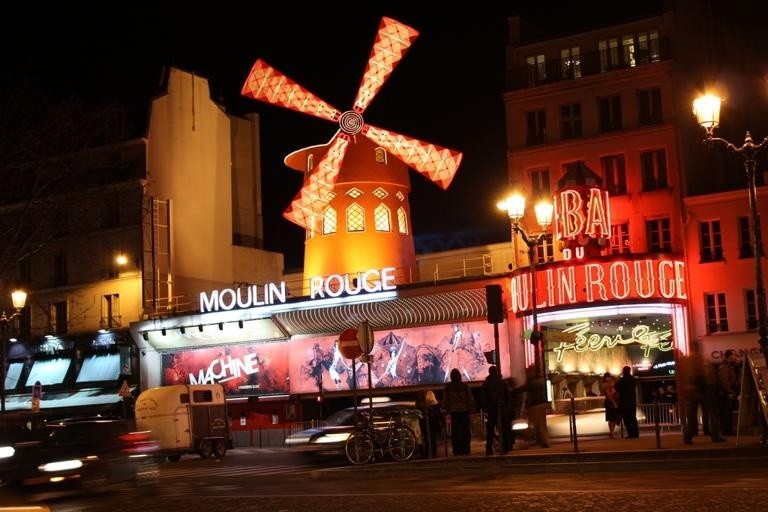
[601,371,620,438]
[426,390,446,460]
[613,365,641,438]
[718,348,738,436]
[509,366,550,448]
[442,369,474,458]
[480,366,511,457]
[681,339,726,443]
[643,384,677,423]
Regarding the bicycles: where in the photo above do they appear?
[345,411,417,465]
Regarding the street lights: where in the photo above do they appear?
[505,192,555,375]
[1,287,28,412]
[693,92,767,361]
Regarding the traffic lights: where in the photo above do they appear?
[486,285,504,323]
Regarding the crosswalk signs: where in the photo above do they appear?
[118,380,130,397]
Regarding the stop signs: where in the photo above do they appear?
[338,329,363,360]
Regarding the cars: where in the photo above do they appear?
[0,416,166,496]
[285,404,424,459]
